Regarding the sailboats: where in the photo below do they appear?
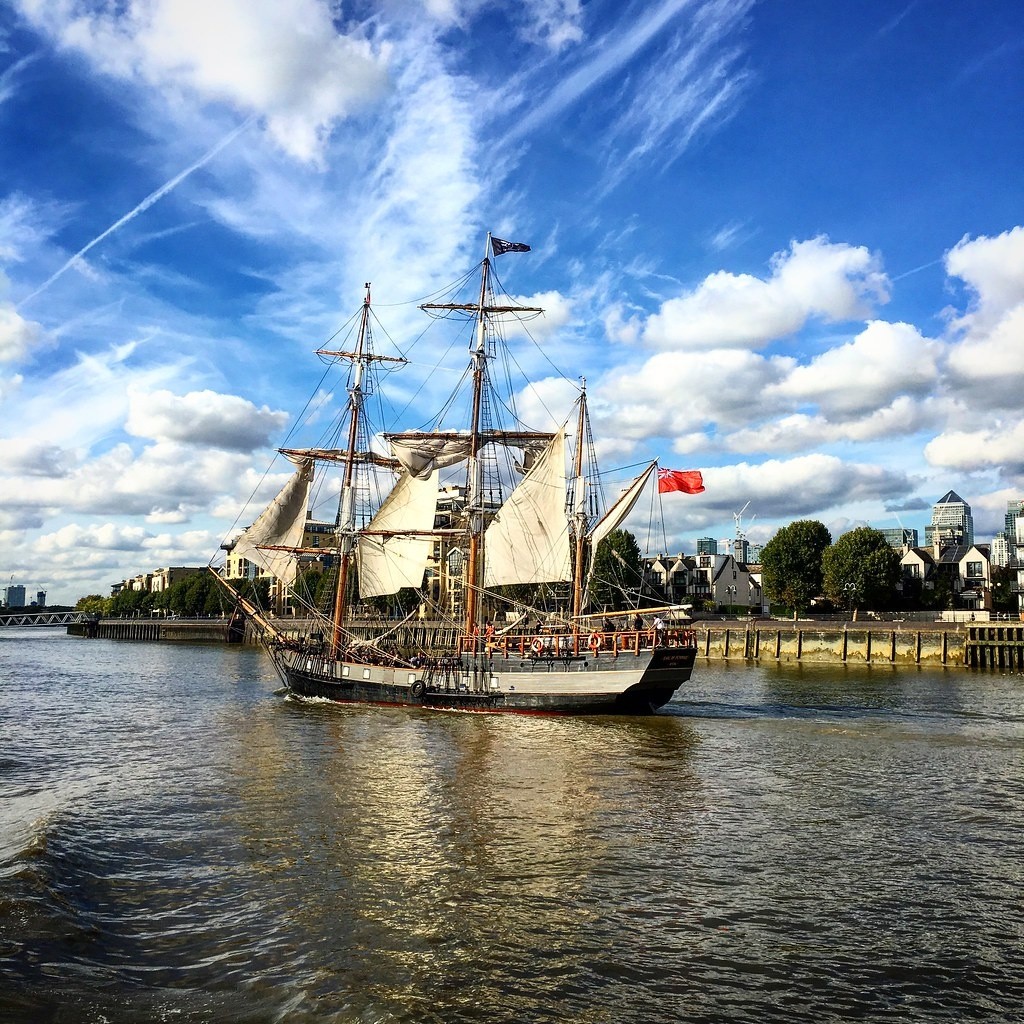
[204,231,703,716]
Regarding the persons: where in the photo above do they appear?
[345,642,425,668]
[970,609,1024,622]
[473,612,669,652]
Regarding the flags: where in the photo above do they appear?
[658,466,705,494]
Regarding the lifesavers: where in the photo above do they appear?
[532,638,544,652]
[588,634,601,648]
